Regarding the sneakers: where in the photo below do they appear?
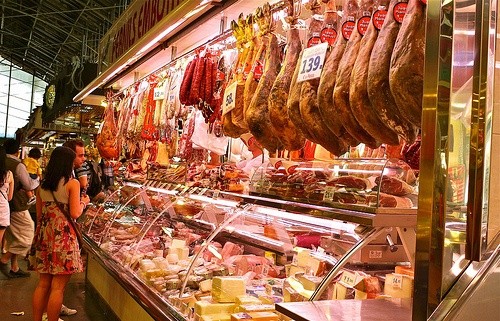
[60,304,77,315]
[42,312,64,321]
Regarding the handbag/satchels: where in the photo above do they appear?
[74,222,83,249]
[88,164,100,196]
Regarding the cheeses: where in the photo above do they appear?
[139,239,414,321]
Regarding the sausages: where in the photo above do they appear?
[179,57,222,122]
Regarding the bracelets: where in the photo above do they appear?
[80,201,86,209]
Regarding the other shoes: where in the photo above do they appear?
[10,267,31,278]
[0,260,16,278]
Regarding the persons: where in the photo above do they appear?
[0,138,90,321]
[231,137,270,176]
